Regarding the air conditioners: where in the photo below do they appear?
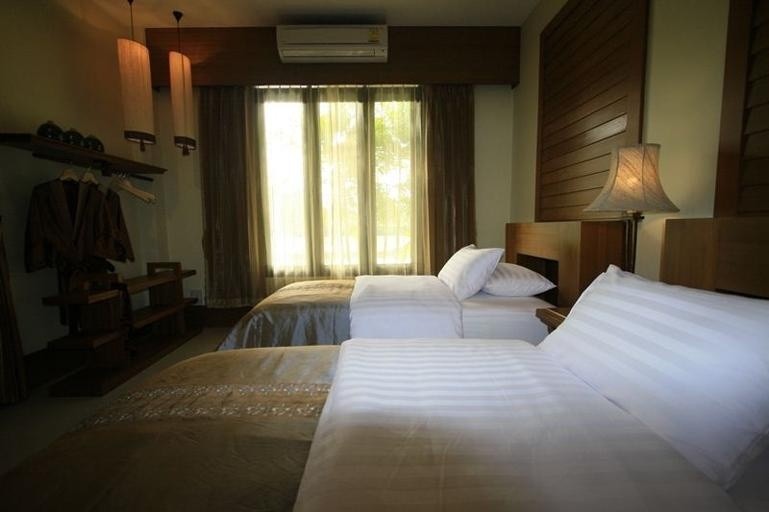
[275,22,390,64]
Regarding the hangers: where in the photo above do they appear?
[50,158,157,204]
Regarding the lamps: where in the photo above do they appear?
[116,0,198,157]
[584,143,678,272]
